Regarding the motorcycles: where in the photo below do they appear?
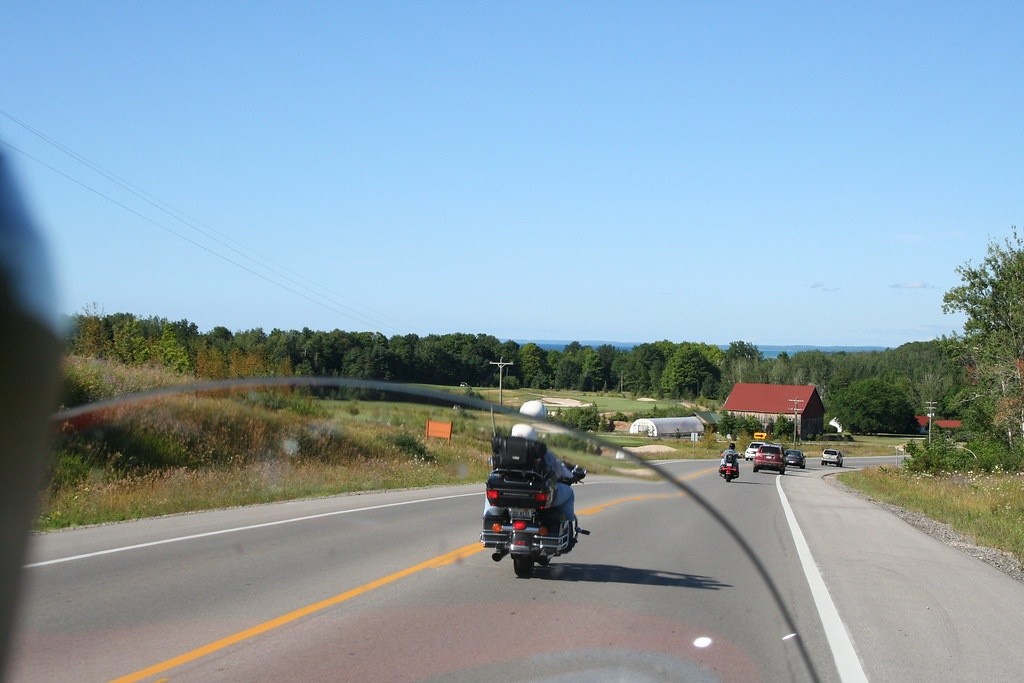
[718,450,742,482]
[479,426,591,579]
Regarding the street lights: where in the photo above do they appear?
[788,399,805,450]
[925,401,937,445]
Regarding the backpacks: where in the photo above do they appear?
[491,435,549,482]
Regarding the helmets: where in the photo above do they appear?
[729,443,735,449]
[511,424,537,442]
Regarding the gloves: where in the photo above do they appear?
[572,468,586,482]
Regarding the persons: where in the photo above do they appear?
[483,399,576,533]
[720,443,739,477]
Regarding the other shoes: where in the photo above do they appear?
[569,537,577,543]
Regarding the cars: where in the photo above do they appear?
[784,449,807,470]
[820,449,845,467]
[744,441,766,461]
[751,444,787,474]
